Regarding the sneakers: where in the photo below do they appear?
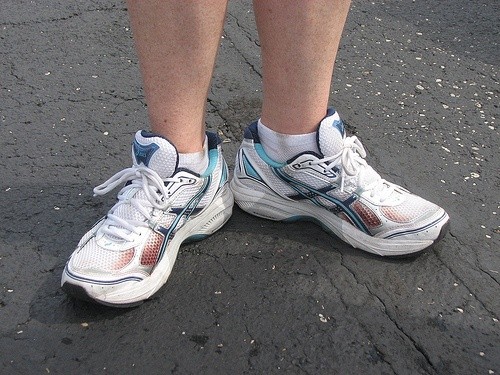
[57,128,234,310]
[230,109,452,260]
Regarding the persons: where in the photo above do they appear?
[60,0,450,308]
